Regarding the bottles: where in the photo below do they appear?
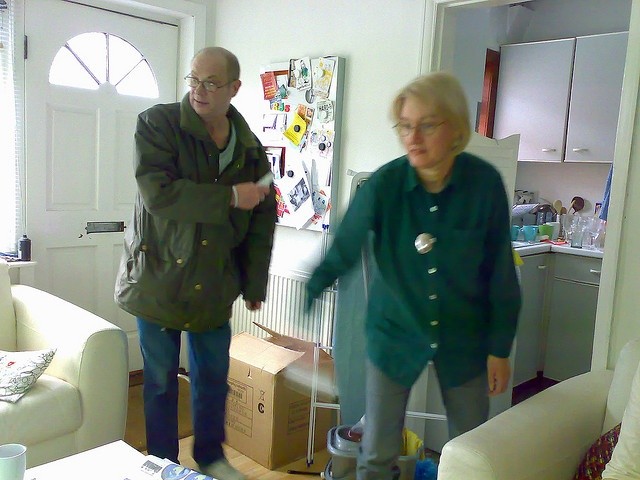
[18,235,31,261]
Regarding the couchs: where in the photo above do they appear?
[436,337,640,479]
[0,259,130,469]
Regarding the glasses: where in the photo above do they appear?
[184,72,234,93]
[392,121,448,136]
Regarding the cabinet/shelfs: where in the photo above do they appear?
[492,28,629,164]
[513,252,550,388]
[543,250,603,383]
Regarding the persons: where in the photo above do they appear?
[301,73,523,479]
[113,47,279,480]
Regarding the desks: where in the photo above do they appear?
[25,438,219,480]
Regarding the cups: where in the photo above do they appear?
[1,443,28,480]
[539,225,554,239]
[558,213,606,249]
[523,225,538,243]
[512,225,521,241]
[546,222,561,239]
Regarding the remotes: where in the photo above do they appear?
[254,171,275,187]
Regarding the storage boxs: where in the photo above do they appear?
[223,321,335,472]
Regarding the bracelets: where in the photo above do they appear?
[231,185,239,208]
